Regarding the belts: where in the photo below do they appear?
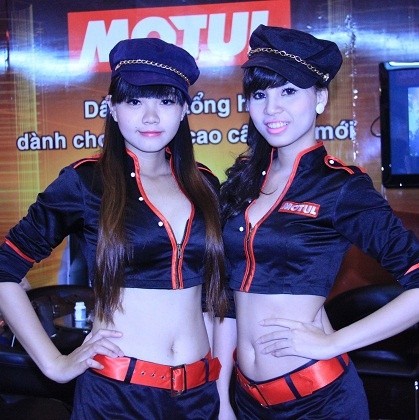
[231,352,351,409]
[89,351,223,397]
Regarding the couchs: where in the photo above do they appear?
[324,283,419,420]
[0,284,95,407]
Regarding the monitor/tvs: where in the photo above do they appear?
[379,60,419,189]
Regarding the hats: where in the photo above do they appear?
[107,38,201,103]
[240,25,343,90]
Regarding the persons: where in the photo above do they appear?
[0,37,225,420]
[212,24,419,420]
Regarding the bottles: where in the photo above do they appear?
[74,301,86,322]
